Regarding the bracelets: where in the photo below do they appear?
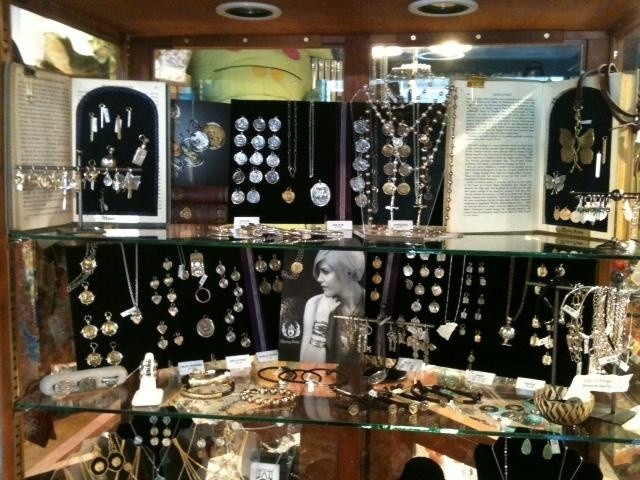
[308,320,330,348]
[180,365,348,408]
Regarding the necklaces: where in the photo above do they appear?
[437,252,470,343]
[369,356,479,410]
[308,100,316,180]
[349,63,457,240]
[497,255,533,348]
[556,280,634,374]
[116,240,147,325]
[485,437,585,480]
[334,289,365,337]
[81,409,298,479]
[285,102,300,181]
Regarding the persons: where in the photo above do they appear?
[299,249,367,362]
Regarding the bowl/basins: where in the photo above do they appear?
[533,385,595,426]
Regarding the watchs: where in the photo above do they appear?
[288,249,304,281]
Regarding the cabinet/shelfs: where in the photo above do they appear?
[8,222,640,479]
